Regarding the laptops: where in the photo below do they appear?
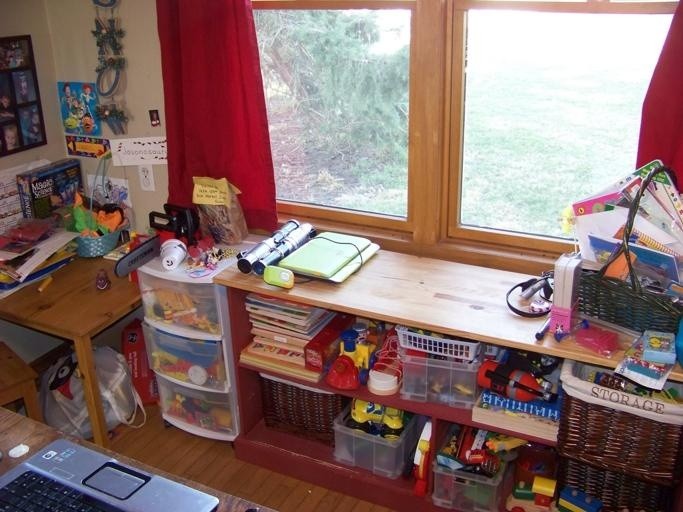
[0,438,219,512]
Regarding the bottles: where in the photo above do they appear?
[476,359,558,405]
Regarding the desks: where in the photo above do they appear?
[0,407,286,512]
[212,234,681,503]
[3,259,141,448]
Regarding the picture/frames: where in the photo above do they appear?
[1,34,49,157]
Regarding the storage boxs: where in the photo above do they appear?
[560,360,683,477]
[40,341,147,441]
[433,455,510,512]
[334,405,425,480]
[558,451,680,511]
[80,234,120,258]
[124,322,161,406]
[400,356,479,410]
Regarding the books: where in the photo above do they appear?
[239,293,337,383]
[614,334,678,390]
[472,396,559,441]
[567,175,683,285]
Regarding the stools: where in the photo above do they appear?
[1,343,42,421]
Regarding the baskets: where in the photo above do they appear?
[555,358,683,511]
[75,230,121,258]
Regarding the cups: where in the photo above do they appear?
[160,239,187,270]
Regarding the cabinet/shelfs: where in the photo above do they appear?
[139,243,253,443]
